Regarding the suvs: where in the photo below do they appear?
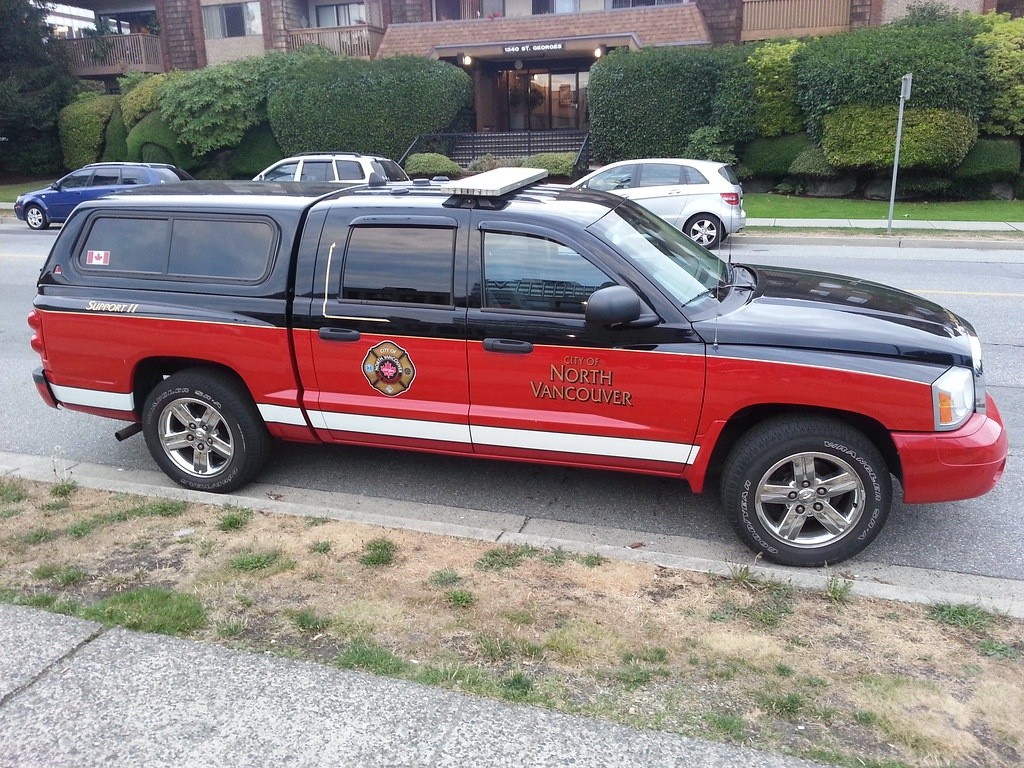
[253,151,415,190]
[569,156,748,250]
[14,161,198,230]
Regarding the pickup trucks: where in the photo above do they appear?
[28,167,1009,568]
